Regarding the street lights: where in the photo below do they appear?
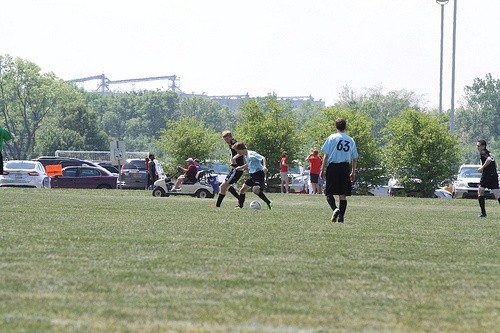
[435,0,450,126]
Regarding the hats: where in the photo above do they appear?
[186,158,193,162]
[314,150,318,154]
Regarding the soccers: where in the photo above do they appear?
[250,200,261,209]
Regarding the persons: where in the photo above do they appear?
[193,159,202,171]
[232,141,273,211]
[175,158,196,189]
[280,151,289,194]
[212,131,245,208]
[477,140,500,217]
[320,119,359,223]
[306,150,321,194]
[145,157,151,191]
[0,125,12,174]
[444,183,452,192]
[148,154,156,190]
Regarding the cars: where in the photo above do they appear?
[117,159,158,190]
[265,160,313,193]
[0,160,46,188]
[31,156,122,175]
[387,167,422,196]
[454,164,491,198]
[50,166,118,190]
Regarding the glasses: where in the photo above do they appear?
[477,145,481,147]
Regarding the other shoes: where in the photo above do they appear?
[480,214,486,217]
[331,208,340,222]
[169,187,176,192]
[235,205,240,209]
[268,200,272,210]
